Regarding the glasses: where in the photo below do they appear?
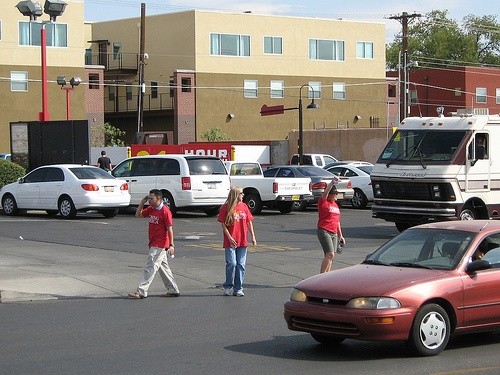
[147,198,157,200]
[239,192,244,195]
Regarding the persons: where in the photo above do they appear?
[91,151,112,172]
[316,177,345,274]
[128,189,180,299]
[217,186,256,297]
[471,238,492,264]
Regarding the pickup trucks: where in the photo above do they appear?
[224,160,315,214]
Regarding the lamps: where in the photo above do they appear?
[355,115,361,119]
[229,114,235,118]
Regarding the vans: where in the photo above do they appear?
[109,154,232,218]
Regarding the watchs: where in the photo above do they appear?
[169,244,175,247]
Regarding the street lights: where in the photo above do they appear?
[297,83,320,165]
[15,0,68,121]
[57,75,81,120]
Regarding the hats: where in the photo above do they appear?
[322,185,338,194]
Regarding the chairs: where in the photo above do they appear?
[442,241,459,259]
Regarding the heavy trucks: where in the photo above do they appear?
[370,113,500,233]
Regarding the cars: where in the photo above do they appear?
[283,220,500,357]
[0,164,131,219]
[263,165,354,211]
[290,153,375,207]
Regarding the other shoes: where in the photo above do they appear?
[161,292,180,297]
[233,291,244,296]
[224,288,231,295]
[128,293,145,298]
